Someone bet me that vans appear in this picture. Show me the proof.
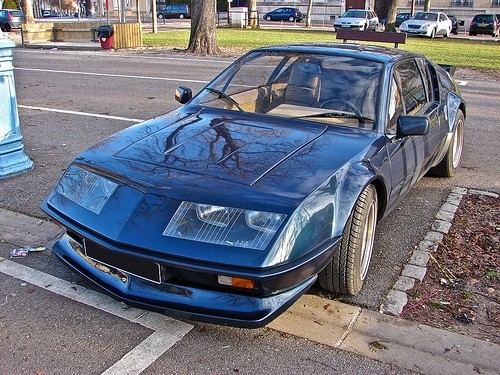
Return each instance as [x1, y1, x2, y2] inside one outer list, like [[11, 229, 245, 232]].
[[157, 4, 191, 19]]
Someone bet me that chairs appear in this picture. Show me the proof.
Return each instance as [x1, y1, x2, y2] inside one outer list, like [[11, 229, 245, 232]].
[[282, 56, 323, 107]]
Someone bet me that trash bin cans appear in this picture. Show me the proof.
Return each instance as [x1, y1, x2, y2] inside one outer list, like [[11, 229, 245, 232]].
[[96, 25, 114, 50]]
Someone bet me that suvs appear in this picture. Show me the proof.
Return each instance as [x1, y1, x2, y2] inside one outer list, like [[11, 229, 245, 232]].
[[469, 13, 500, 37]]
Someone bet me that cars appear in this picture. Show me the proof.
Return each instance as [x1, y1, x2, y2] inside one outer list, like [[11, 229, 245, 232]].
[[381, 13, 412, 27], [447, 15, 458, 35], [40, 42, 466, 329], [43, 11, 81, 18], [0, 9, 23, 32], [333, 9, 379, 32], [400, 12, 452, 39], [263, 7, 303, 22]]
